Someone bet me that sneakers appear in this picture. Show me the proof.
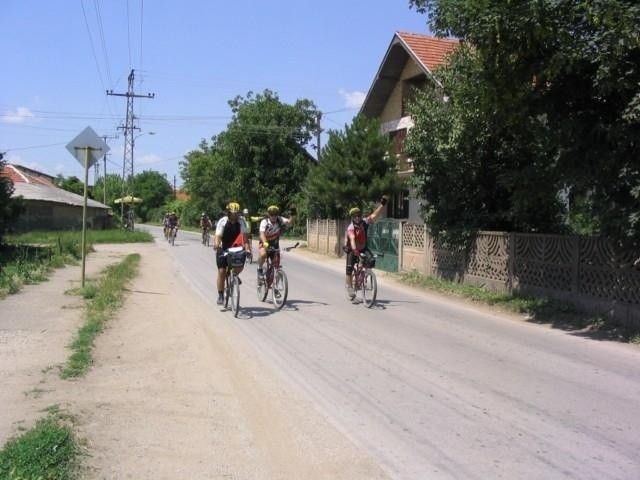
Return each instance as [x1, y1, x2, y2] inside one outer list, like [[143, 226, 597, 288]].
[[257, 269, 264, 279], [217, 290, 224, 304]]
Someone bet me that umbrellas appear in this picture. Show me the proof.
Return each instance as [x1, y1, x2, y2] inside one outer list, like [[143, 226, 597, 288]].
[[114, 195, 142, 204]]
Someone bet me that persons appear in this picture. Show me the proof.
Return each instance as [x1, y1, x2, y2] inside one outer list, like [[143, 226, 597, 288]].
[[200, 212, 213, 241], [239, 208, 265, 236], [344, 194, 390, 297], [213, 203, 253, 305], [162, 212, 180, 238], [256, 203, 297, 298]]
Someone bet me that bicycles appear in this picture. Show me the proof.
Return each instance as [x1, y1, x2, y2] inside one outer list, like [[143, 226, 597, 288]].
[[159, 222, 180, 247], [200, 225, 213, 248], [257, 240, 302, 307], [341, 247, 384, 310], [216, 242, 253, 318]]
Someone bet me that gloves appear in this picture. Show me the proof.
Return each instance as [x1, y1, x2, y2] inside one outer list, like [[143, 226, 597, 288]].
[[380, 195, 389, 205]]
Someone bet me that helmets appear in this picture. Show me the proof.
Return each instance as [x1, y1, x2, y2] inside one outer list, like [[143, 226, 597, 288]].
[[349, 207, 361, 216], [226, 202, 240, 214], [268, 205, 280, 214]]
